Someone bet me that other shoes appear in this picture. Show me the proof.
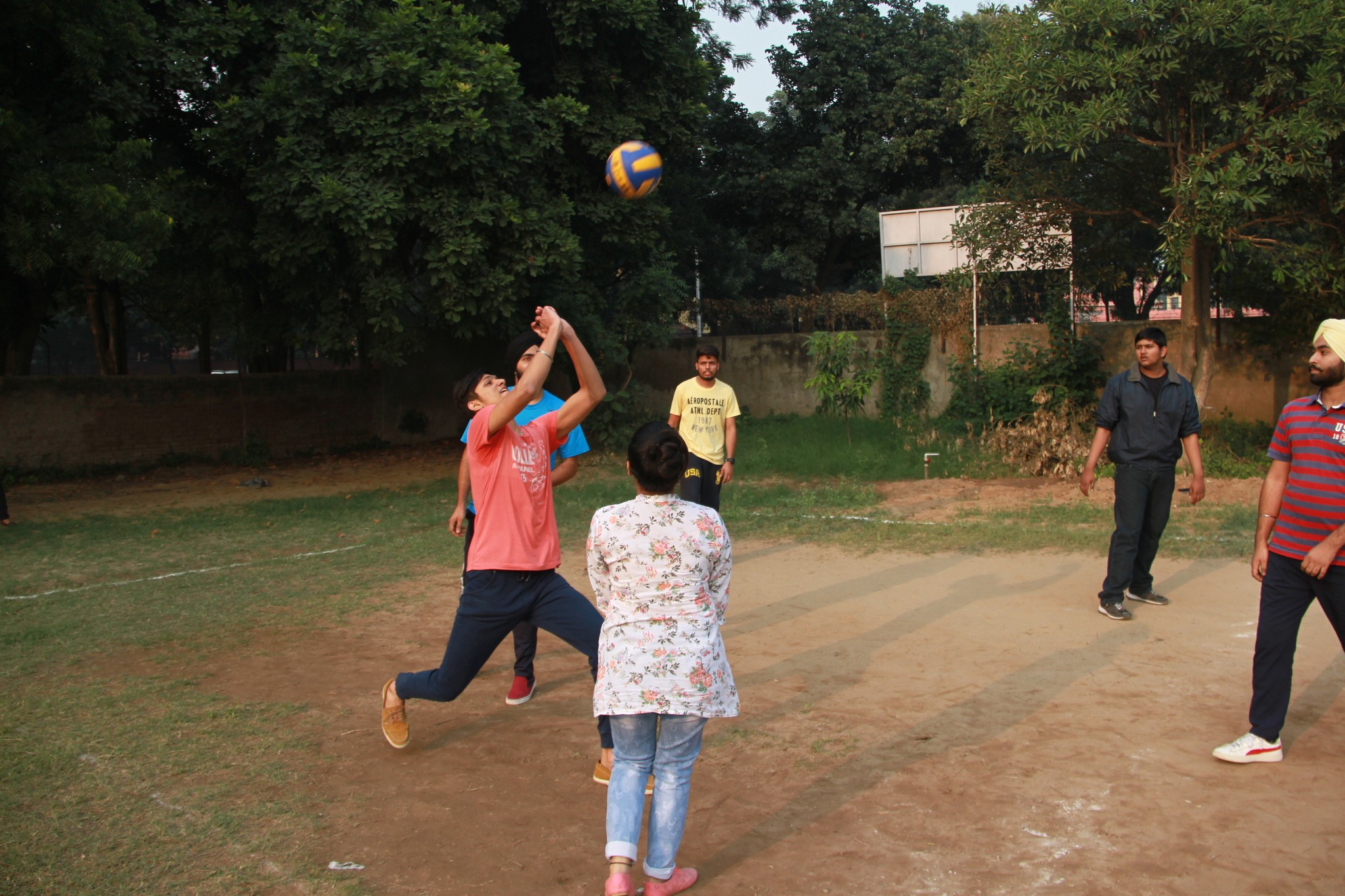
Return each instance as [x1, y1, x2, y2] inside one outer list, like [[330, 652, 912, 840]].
[[604, 872, 635, 895], [645, 867, 697, 896]]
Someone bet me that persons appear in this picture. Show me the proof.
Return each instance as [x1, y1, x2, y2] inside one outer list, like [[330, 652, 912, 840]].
[[586, 420, 742, 896], [447, 331, 590, 706], [379, 304, 665, 797], [1208, 315, 1345, 764], [1078, 326, 1206, 621], [668, 345, 742, 511]]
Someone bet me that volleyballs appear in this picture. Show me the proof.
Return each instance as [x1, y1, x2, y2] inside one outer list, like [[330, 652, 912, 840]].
[[605, 141, 663, 198]]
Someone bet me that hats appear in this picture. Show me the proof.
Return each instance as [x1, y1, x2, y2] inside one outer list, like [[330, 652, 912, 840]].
[[1313, 318, 1345, 362]]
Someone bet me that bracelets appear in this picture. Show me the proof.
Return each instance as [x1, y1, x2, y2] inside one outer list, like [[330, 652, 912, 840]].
[[1258, 514, 1276, 519], [536, 350, 554, 363]]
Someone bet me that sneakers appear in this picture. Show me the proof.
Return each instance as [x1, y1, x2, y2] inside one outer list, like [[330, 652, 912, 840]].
[[381, 678, 410, 749], [505, 674, 536, 704], [1126, 590, 1169, 605], [1212, 732, 1283, 764], [1098, 602, 1131, 620], [592, 760, 654, 794]]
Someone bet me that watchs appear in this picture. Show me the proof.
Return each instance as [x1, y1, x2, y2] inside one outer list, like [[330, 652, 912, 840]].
[[725, 457, 735, 465]]
